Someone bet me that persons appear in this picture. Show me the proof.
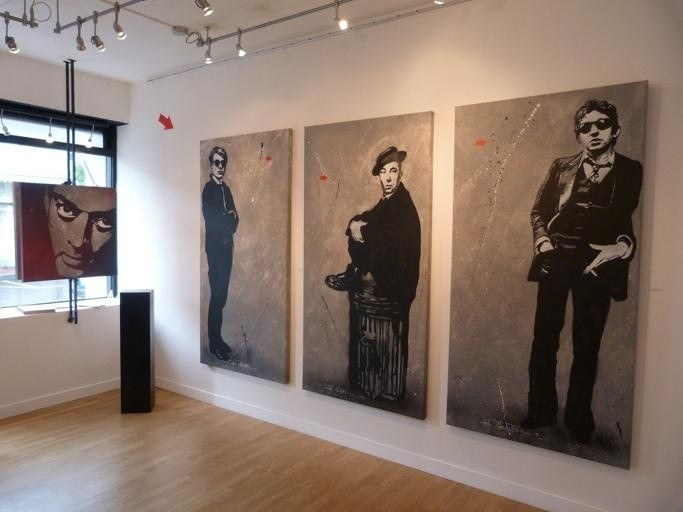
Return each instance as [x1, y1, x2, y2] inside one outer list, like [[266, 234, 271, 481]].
[[324, 145, 422, 311], [201, 145, 239, 364], [515, 99, 644, 439], [40, 185, 116, 280]]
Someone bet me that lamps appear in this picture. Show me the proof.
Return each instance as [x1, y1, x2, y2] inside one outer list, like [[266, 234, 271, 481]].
[[113, 2, 126, 40], [236, 30, 245, 57], [0, 109, 95, 149], [197, 33, 204, 47], [334, 3, 349, 30], [195, 1, 214, 17], [77, 15, 86, 52], [91, 11, 106, 52], [5, 11, 19, 53], [204, 37, 212, 65]]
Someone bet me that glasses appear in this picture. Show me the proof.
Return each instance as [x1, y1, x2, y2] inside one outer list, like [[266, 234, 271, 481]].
[[211, 160, 225, 166], [577, 119, 611, 135]]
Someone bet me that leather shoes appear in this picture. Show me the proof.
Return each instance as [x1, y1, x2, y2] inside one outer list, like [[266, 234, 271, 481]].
[[216, 341, 231, 361], [325, 264, 363, 290]]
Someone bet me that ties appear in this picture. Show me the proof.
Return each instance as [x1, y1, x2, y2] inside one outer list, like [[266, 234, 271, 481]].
[[589, 164, 600, 183]]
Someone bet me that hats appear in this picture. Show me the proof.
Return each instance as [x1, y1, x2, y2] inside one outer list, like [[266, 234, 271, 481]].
[[372, 147, 406, 176]]
